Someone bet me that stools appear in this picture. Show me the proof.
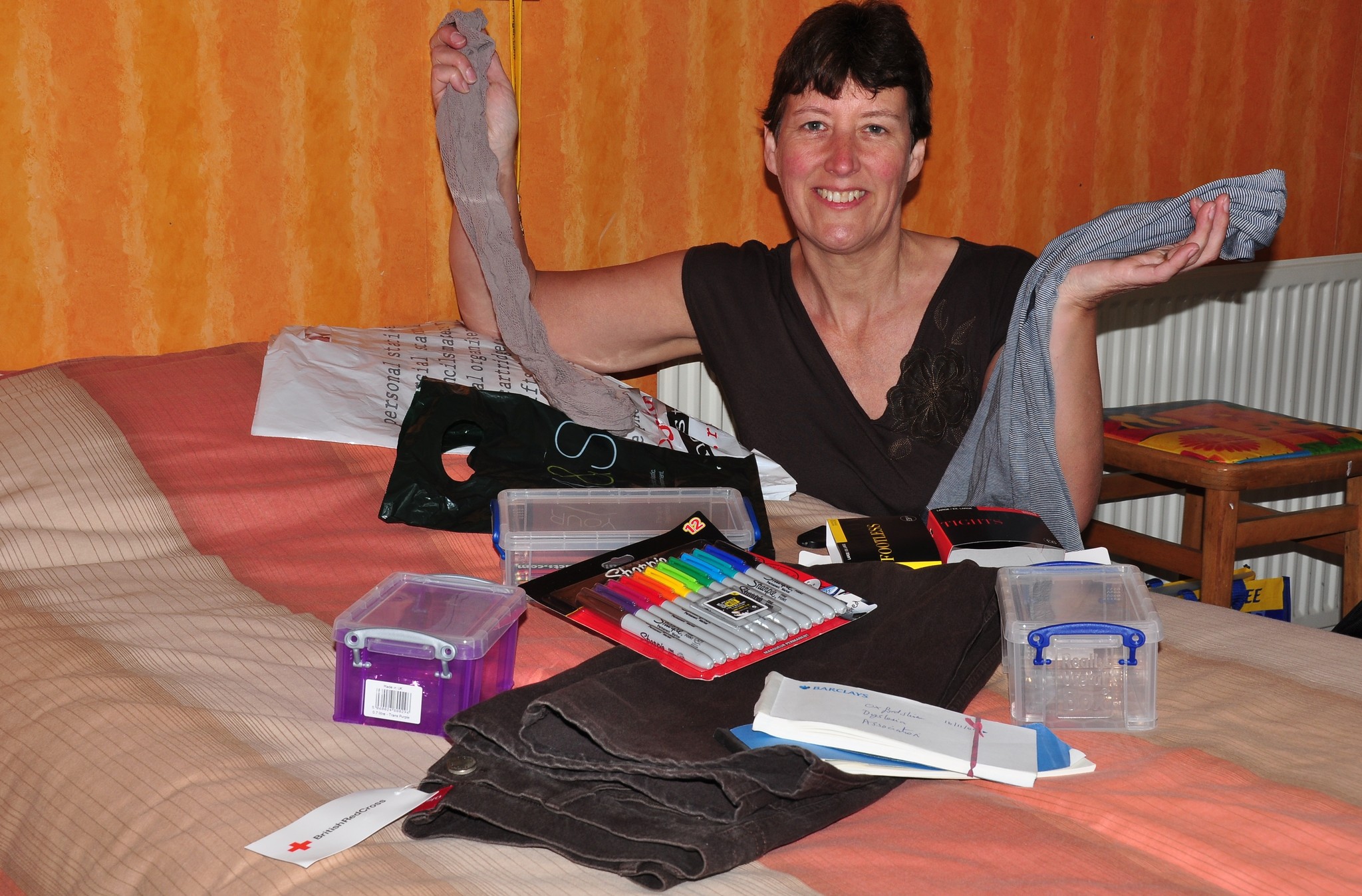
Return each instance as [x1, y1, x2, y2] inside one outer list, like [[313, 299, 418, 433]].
[[1081, 400, 1361, 620]]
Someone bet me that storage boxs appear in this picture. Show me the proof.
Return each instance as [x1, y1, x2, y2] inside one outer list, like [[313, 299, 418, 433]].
[[996, 561, 1163, 731], [823, 514, 944, 570], [332, 572, 529, 737], [929, 506, 1067, 568], [488, 486, 764, 589]]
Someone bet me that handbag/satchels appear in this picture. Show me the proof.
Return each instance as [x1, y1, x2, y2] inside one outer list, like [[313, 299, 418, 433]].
[[378, 375, 775, 559], [1145, 566, 1290, 630], [250, 320, 799, 502]]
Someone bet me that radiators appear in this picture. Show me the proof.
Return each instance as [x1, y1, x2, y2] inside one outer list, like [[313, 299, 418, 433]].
[[655, 252, 1362, 627]]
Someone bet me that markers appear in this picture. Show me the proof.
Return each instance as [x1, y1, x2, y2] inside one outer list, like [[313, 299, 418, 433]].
[[574, 540, 849, 668]]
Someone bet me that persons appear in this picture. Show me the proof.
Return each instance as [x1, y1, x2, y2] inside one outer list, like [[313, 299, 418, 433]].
[[429, 0, 1229, 533]]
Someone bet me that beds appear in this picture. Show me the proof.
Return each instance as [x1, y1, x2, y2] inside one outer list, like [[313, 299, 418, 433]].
[[0, 338, 1362, 896]]
